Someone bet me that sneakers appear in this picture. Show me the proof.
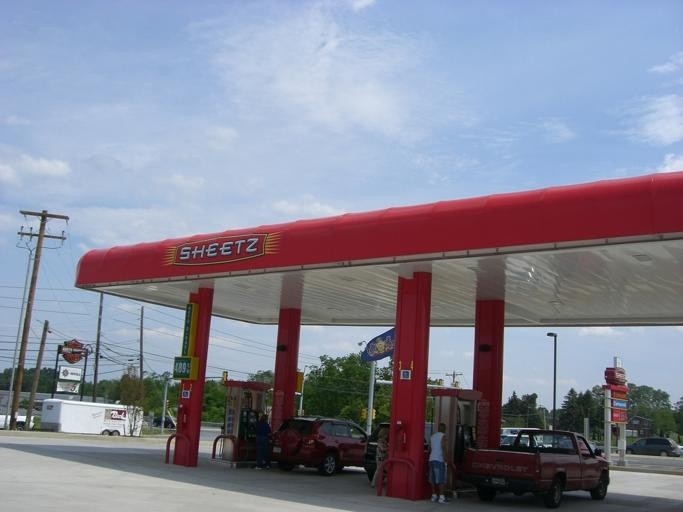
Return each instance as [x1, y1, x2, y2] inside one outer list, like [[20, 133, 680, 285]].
[[431, 493, 448, 504]]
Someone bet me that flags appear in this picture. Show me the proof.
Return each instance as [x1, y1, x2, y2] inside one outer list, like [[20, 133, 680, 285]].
[[361, 327, 395, 362]]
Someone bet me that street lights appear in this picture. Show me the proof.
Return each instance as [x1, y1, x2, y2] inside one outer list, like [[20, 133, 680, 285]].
[[548, 333, 556, 448], [358, 340, 375, 436]]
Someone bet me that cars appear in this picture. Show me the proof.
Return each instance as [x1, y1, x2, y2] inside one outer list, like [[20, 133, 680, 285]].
[[153, 415, 177, 428], [626, 437, 681, 457]]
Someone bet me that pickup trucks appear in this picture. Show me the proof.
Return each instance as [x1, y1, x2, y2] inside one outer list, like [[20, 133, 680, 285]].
[[0, 415, 36, 430]]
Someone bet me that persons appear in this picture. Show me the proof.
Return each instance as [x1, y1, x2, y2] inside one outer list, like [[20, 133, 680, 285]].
[[427, 423, 448, 504], [370, 427, 390, 488], [254, 414, 272, 470]]
